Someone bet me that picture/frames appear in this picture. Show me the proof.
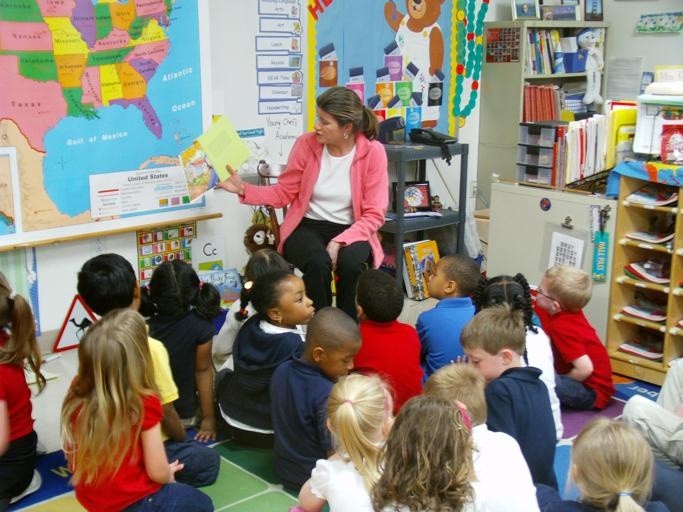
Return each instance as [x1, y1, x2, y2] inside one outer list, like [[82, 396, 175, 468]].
[[392, 180, 432, 213], [511, 0, 541, 20], [584, 0, 603, 21]]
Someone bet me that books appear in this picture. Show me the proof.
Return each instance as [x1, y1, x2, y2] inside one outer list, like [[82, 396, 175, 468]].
[[618, 335, 664, 361], [625, 230, 674, 245], [400, 238, 441, 300], [631, 63, 683, 167], [524, 29, 566, 76], [622, 258, 670, 287], [177, 114, 252, 205], [550, 101, 642, 188], [619, 296, 667, 323], [524, 83, 561, 121], [625, 181, 678, 206]]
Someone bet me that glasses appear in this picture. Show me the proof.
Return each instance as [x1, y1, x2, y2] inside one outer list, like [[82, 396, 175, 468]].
[[535, 286, 564, 311]]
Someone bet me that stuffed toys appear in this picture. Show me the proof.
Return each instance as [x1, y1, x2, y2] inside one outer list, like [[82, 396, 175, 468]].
[[578, 30, 604, 105]]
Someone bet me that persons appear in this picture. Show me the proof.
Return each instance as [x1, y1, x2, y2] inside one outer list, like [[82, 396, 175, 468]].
[[474, 273, 565, 443], [268, 306, 364, 491], [58, 307, 221, 512], [415, 253, 482, 387], [0, 268, 47, 512], [214, 85, 389, 326], [211, 248, 308, 371], [533, 416, 669, 512], [288, 373, 396, 512], [75, 252, 221, 488], [348, 268, 425, 419], [368, 394, 482, 512], [138, 257, 221, 444], [424, 362, 541, 512], [459, 302, 561, 493], [215, 269, 316, 450], [530, 264, 615, 411], [621, 357, 683, 471], [518, 4, 535, 17]]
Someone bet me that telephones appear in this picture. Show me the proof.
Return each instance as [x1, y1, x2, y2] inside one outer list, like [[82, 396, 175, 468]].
[[409, 128, 458, 166]]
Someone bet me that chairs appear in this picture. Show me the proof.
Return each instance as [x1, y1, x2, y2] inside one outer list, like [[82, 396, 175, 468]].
[[260, 175, 298, 274]]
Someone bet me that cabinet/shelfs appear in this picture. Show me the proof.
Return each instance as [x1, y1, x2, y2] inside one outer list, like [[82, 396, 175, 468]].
[[377, 140, 472, 293], [481, 18, 610, 195], [605, 158, 683, 388]]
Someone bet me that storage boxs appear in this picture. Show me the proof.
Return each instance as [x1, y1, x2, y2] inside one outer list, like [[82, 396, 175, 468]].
[[516, 121, 558, 148], [516, 162, 553, 186], [515, 143, 556, 169]]
